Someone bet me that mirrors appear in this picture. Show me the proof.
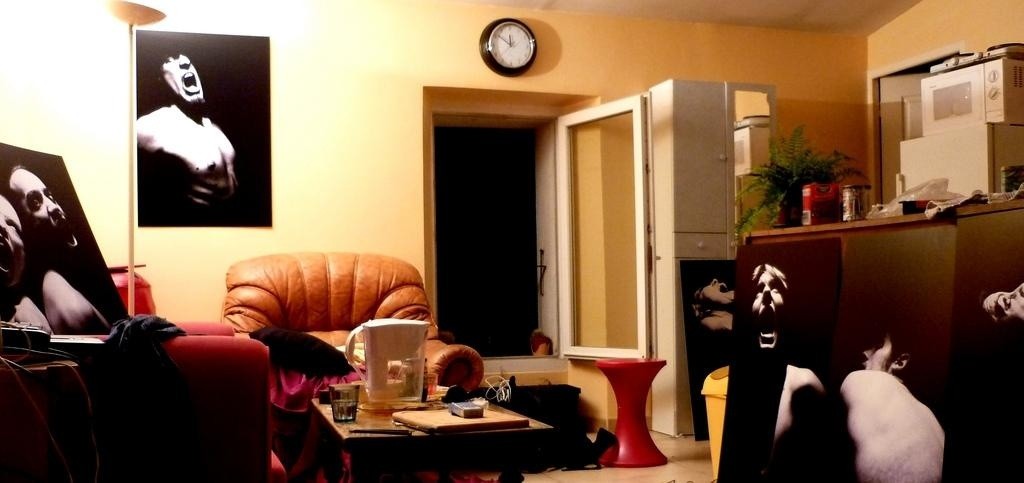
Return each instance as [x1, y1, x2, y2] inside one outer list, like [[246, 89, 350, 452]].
[[733, 88, 770, 253]]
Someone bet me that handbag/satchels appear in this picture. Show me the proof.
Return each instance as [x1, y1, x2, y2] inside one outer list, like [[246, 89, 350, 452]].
[[469, 382, 592, 472]]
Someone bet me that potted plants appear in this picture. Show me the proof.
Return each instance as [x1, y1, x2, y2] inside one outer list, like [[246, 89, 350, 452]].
[[726, 121, 867, 244]]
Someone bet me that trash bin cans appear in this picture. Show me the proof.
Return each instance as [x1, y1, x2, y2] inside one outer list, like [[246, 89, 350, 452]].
[[701, 365, 729, 481]]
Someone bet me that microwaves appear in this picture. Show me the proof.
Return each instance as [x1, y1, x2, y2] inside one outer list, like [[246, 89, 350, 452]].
[[734, 127, 772, 175], [919, 59, 1024, 137]]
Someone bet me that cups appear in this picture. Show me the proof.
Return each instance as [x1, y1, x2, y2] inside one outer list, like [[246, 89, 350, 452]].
[[328, 384, 360, 422]]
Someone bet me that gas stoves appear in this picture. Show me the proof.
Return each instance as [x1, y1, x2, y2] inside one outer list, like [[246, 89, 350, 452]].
[[928, 42, 1024, 73], [735, 114, 771, 131]]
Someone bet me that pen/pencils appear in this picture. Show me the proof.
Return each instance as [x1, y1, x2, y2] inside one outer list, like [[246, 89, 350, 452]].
[[349, 429, 412, 435]]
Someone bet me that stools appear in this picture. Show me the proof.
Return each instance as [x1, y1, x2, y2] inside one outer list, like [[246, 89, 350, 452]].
[[593, 355, 669, 467]]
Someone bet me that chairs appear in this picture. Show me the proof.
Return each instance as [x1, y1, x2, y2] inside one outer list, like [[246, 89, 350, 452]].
[[111, 268, 272, 476], [226, 252, 483, 440]]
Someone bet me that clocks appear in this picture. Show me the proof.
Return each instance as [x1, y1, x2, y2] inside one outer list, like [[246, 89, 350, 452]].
[[481, 18, 537, 77]]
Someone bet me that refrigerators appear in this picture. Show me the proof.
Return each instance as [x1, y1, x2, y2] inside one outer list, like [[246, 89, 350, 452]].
[[735, 172, 774, 232], [896, 124, 1024, 203]]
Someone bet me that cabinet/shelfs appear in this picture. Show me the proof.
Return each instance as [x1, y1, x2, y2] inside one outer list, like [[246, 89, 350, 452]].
[[650, 78, 777, 438]]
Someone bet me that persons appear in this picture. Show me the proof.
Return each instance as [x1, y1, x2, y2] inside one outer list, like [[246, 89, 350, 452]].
[[980, 282, 1024, 329], [6, 164, 111, 335], [749, 263, 827, 483], [691, 278, 735, 331], [137, 47, 239, 226], [0, 193, 55, 336], [837, 333, 944, 483]]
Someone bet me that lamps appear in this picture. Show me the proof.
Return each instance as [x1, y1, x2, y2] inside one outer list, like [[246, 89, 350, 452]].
[[103, 0, 165, 319]]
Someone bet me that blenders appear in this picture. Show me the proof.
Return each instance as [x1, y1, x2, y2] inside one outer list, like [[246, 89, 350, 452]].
[[344, 318, 430, 408]]
